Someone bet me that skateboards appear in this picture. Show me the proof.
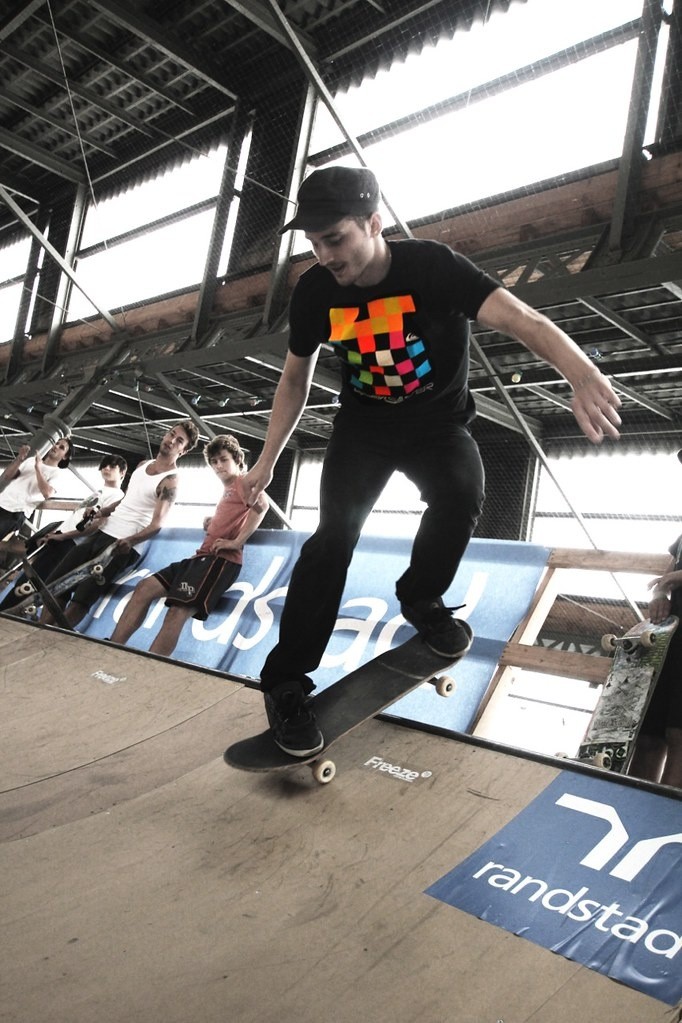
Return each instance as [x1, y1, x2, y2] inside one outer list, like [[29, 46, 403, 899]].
[[0, 542, 51, 582], [574, 613, 676, 779], [223, 614, 474, 785], [5, 540, 120, 617], [11, 553, 75, 634]]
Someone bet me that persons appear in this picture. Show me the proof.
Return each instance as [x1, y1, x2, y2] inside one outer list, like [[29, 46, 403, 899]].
[[38, 419, 199, 630], [103, 434, 269, 657], [0, 455, 127, 615], [627, 534, 682, 788], [0, 438, 74, 542], [238, 167, 622, 759]]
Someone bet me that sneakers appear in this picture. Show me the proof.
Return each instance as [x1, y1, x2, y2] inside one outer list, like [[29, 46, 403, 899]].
[[264, 683, 324, 757], [400, 596, 472, 658]]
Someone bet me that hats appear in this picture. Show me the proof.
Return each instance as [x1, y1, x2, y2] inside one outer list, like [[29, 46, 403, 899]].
[[278, 166, 380, 236]]
